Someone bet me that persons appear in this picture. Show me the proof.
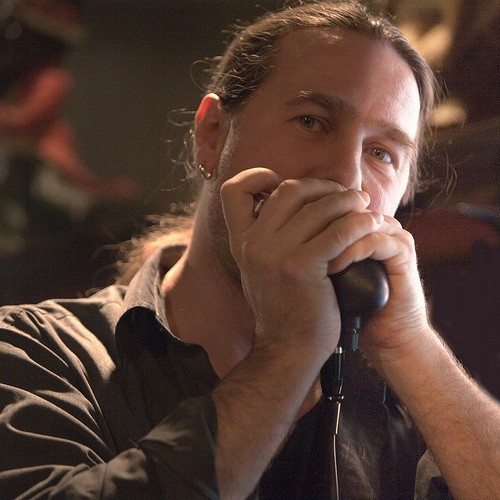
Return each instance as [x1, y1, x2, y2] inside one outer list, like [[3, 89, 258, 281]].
[[0, 2, 499, 500]]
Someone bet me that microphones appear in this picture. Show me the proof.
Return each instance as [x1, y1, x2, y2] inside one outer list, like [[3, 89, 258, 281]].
[[327, 257, 390, 405]]
